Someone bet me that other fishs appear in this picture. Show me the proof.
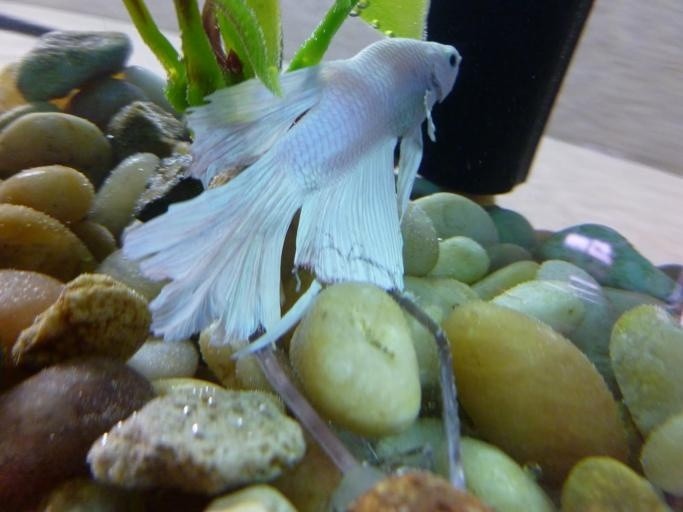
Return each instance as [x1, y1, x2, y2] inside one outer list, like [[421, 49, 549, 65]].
[[120, 37, 463, 350]]
